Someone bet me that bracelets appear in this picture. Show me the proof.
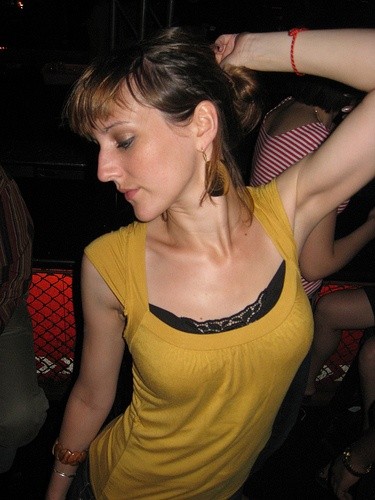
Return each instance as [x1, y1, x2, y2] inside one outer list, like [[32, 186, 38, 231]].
[[52, 469, 77, 480], [288, 28, 308, 75], [52, 437, 87, 466]]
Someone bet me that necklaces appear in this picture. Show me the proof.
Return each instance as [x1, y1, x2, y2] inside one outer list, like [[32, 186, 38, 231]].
[[314, 107, 321, 123]]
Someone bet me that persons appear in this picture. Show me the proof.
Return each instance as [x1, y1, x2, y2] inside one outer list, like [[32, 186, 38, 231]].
[[0, 19, 375, 500]]
